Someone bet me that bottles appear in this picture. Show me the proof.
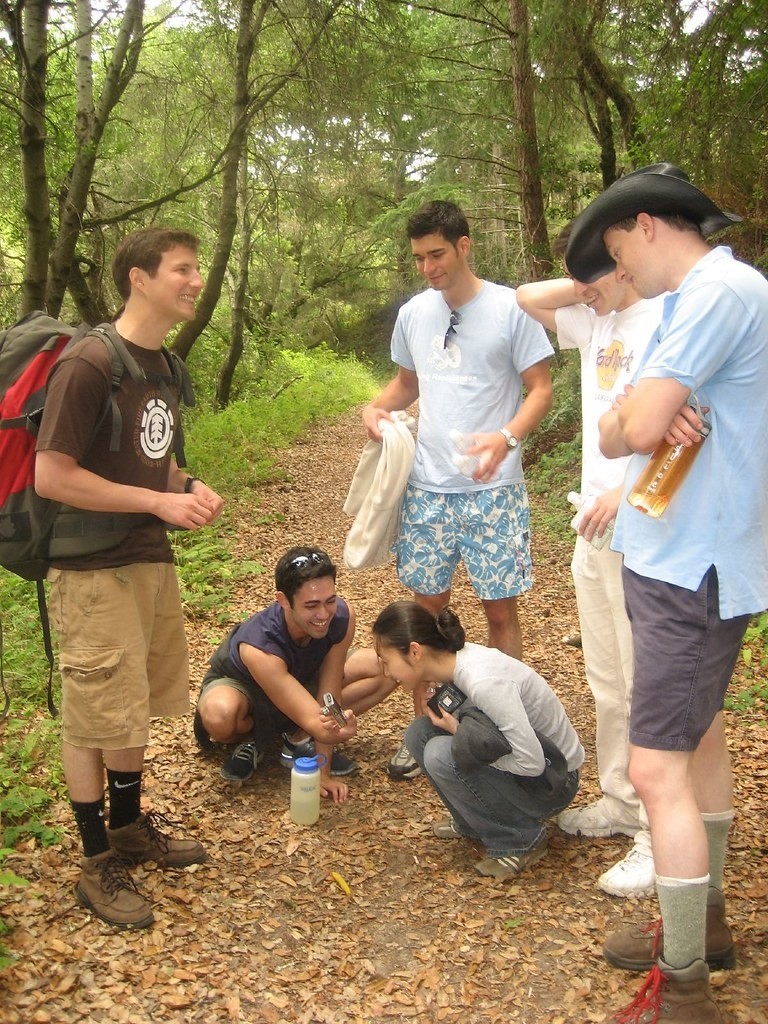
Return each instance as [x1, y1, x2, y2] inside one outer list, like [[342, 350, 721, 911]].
[[448, 430, 480, 458], [567, 492, 615, 526], [451, 454, 504, 482], [290, 753, 327, 825], [627, 395, 711, 518]]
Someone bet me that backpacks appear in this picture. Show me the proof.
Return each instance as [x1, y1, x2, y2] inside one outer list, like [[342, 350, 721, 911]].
[[0, 310, 195, 580]]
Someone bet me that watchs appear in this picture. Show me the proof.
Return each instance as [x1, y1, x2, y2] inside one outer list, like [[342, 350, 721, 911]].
[[500, 427, 518, 449]]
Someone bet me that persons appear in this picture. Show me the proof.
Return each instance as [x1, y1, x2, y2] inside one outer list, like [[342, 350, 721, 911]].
[[564, 161, 768, 1024], [196, 545, 401, 802], [35, 231, 226, 931], [516, 217, 676, 899], [363, 202, 556, 780], [372, 601, 585, 878]]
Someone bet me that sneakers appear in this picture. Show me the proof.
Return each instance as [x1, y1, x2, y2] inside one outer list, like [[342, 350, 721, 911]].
[[74, 848, 154, 930], [598, 849, 656, 898], [106, 809, 207, 866], [433, 819, 462, 838], [221, 742, 264, 782], [599, 955, 723, 1024], [557, 796, 642, 838], [387, 742, 422, 779], [281, 732, 362, 776], [474, 835, 549, 877], [603, 887, 736, 970]]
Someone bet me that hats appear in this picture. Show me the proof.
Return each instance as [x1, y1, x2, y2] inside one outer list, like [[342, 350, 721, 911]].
[[565, 163, 743, 284]]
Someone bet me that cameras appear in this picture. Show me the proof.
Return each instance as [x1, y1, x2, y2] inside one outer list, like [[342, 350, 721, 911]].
[[427, 681, 467, 718], [322, 693, 346, 729]]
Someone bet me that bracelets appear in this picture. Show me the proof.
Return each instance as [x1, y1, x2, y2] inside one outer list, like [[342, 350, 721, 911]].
[[185, 477, 207, 493]]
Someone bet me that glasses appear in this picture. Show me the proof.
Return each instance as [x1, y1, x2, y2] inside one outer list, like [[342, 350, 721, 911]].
[[442, 310, 462, 350], [282, 552, 328, 582]]
